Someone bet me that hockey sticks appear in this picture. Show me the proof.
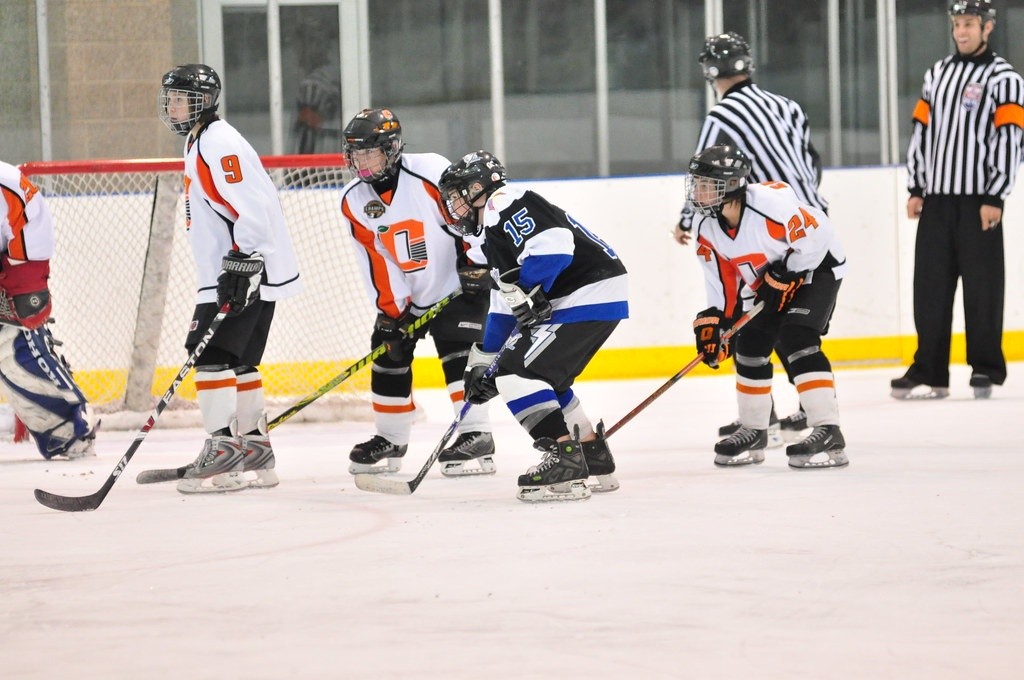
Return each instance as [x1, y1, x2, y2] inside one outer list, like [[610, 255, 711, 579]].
[[134, 289, 464, 484], [602, 302, 770, 437], [352, 313, 526, 496], [34, 300, 233, 514]]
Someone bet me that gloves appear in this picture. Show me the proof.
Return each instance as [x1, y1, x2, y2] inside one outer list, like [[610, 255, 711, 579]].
[[216, 250, 263, 316], [752, 259, 810, 316], [492, 269, 552, 326], [693, 306, 736, 369], [464, 342, 499, 404], [0, 254, 52, 330]]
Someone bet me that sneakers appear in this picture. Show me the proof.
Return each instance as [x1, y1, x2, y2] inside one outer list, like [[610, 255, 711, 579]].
[[438, 432, 496, 476], [349, 435, 408, 474], [243, 412, 280, 487], [176, 416, 244, 493], [580, 419, 621, 492], [786, 424, 850, 468], [779, 412, 809, 431], [714, 425, 768, 465], [970, 373, 992, 398], [60, 437, 96, 456], [517, 424, 592, 501], [719, 410, 785, 448], [890, 363, 950, 399]]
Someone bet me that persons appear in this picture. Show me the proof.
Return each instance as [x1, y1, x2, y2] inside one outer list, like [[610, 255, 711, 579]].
[[285, 17, 343, 154], [337, 107, 495, 464], [0, 160, 102, 461], [159, 63, 300, 479], [674, 32, 845, 456], [438, 149, 630, 487], [889, 0, 1024, 389]]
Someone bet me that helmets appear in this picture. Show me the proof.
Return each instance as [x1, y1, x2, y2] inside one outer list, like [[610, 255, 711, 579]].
[[686, 145, 752, 216], [948, 0, 997, 24], [343, 108, 406, 184], [162, 64, 221, 136], [698, 30, 755, 84], [439, 150, 511, 236]]
